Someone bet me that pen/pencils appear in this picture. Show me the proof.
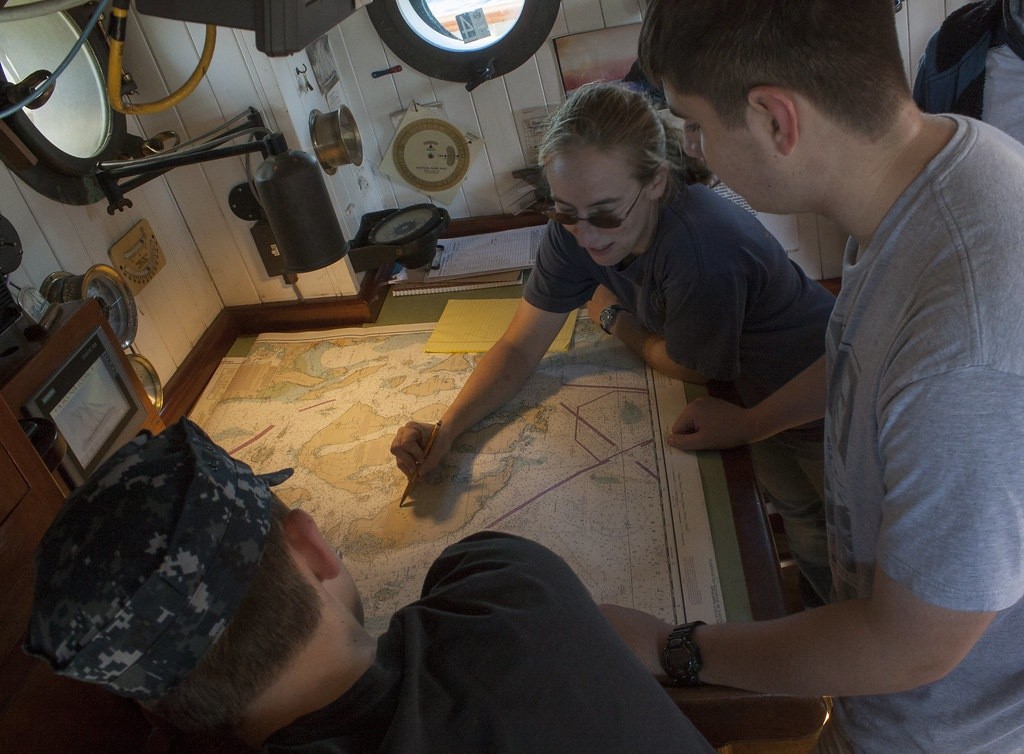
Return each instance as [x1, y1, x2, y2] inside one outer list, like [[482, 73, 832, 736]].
[[399, 419, 443, 506]]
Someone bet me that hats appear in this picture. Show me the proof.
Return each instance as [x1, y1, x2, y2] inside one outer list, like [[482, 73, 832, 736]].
[[24, 417, 295, 702]]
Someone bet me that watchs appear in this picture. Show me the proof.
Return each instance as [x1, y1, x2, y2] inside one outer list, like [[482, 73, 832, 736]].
[[599, 304, 632, 335], [660, 622, 708, 690]]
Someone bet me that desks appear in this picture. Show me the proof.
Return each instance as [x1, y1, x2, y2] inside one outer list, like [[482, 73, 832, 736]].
[[186, 220, 828, 754]]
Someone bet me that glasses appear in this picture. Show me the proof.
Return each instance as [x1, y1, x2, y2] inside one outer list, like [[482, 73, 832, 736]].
[[540, 179, 643, 229]]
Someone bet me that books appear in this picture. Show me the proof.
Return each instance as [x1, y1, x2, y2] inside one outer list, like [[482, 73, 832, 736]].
[[388, 224, 548, 297]]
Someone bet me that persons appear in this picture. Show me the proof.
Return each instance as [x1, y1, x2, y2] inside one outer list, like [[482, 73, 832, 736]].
[[390, 82, 842, 617], [592, 1, 1024, 753], [912, 1, 1024, 143], [14, 418, 725, 754]]
